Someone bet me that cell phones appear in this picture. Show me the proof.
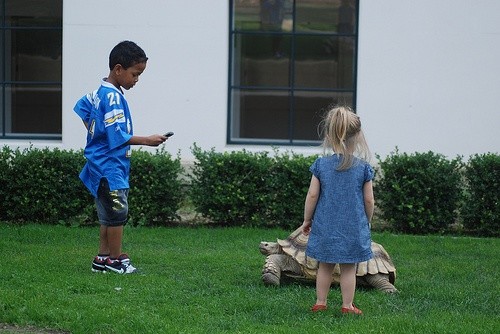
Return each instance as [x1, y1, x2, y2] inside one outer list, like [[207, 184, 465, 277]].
[[164, 132, 174, 137]]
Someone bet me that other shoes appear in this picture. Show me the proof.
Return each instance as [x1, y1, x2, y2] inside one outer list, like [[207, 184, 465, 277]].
[[342, 303, 363, 314], [312, 305, 326, 310]]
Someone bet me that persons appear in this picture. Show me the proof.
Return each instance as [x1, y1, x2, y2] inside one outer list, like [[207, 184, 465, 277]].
[[73, 40, 168, 275], [301, 106, 375, 315]]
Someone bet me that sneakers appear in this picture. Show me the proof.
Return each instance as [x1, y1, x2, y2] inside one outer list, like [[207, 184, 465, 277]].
[[91, 255, 110, 272], [103, 254, 136, 274]]
